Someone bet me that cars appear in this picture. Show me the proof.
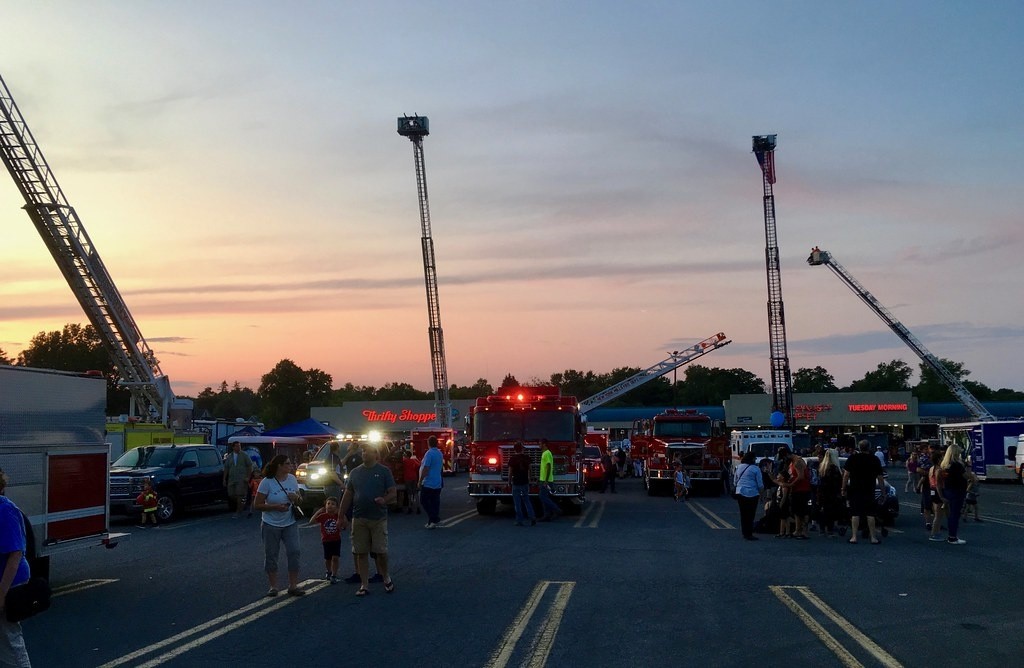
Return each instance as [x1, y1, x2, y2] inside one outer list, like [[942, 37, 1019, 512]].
[[776, 456, 900, 517]]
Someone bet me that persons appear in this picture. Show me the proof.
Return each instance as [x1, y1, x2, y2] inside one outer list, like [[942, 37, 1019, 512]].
[[674, 463, 693, 503], [507, 443, 538, 527], [137, 481, 160, 529], [307, 434, 444, 597], [536, 439, 561, 522], [248, 468, 263, 518], [599, 444, 643, 494], [223, 441, 253, 518], [0, 468, 32, 668], [253, 454, 305, 596], [733, 438, 983, 544]]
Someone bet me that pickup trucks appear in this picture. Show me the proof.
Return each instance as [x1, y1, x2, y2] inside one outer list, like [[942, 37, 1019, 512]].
[[292, 435, 444, 515], [109, 444, 227, 524]]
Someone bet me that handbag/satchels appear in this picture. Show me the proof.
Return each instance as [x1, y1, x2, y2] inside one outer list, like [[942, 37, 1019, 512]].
[[4, 555, 52, 622], [936, 469, 956, 505], [292, 506, 305, 521], [731, 487, 739, 500]]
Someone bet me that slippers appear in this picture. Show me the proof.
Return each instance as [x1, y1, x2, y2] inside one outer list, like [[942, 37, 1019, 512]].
[[355, 586, 368, 597], [384, 577, 394, 593]]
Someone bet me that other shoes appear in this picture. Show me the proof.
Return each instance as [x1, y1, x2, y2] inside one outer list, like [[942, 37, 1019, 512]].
[[773, 532, 792, 539], [288, 584, 306, 596], [424, 522, 436, 529], [344, 573, 360, 583], [791, 534, 808, 542], [151, 523, 159, 528], [743, 533, 758, 540], [871, 539, 882, 544], [325, 570, 332, 580], [535, 516, 551, 522], [368, 574, 384, 583], [847, 537, 857, 544], [974, 518, 983, 522], [947, 536, 966, 544], [963, 519, 967, 522], [926, 523, 933, 529], [929, 534, 945, 541], [267, 587, 278, 597], [136, 523, 146, 529], [330, 575, 341, 584]]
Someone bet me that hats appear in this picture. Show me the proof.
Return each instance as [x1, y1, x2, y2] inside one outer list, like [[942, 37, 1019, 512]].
[[360, 443, 378, 453], [778, 448, 791, 460], [877, 446, 881, 448]]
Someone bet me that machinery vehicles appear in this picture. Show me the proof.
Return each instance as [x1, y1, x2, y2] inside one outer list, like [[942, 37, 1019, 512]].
[[751, 131, 811, 450], [396, 112, 471, 474]]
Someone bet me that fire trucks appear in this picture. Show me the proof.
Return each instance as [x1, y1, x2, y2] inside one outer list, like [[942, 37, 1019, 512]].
[[630, 408, 733, 496], [581, 426, 615, 482], [463, 332, 733, 514]]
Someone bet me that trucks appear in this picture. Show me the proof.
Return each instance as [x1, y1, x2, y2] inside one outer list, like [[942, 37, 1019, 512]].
[[731, 427, 793, 474]]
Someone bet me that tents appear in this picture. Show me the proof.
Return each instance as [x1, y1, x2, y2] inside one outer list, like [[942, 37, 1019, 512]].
[[216, 418, 341, 452]]
